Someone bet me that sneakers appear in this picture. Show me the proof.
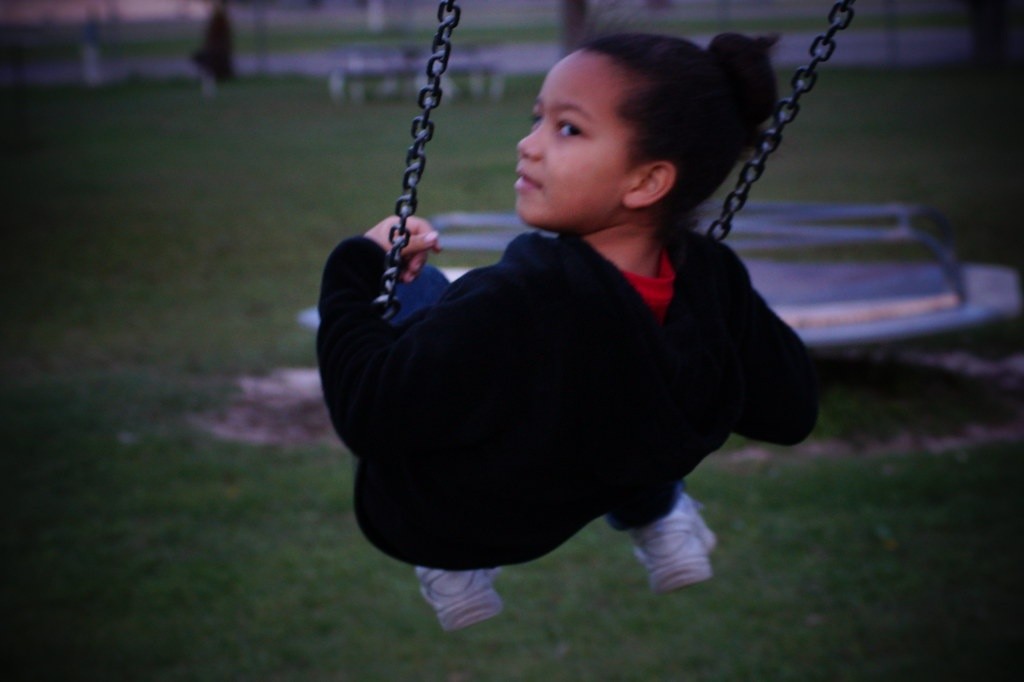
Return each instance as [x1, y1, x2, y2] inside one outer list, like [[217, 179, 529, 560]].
[[415, 566, 502, 633], [630, 492, 716, 593]]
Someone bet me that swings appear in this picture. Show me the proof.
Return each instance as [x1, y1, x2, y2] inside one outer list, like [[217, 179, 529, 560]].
[[372, 0, 853, 511]]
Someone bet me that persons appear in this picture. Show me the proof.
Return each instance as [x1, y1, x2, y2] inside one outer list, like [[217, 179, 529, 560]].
[[316, 33, 822, 630]]
